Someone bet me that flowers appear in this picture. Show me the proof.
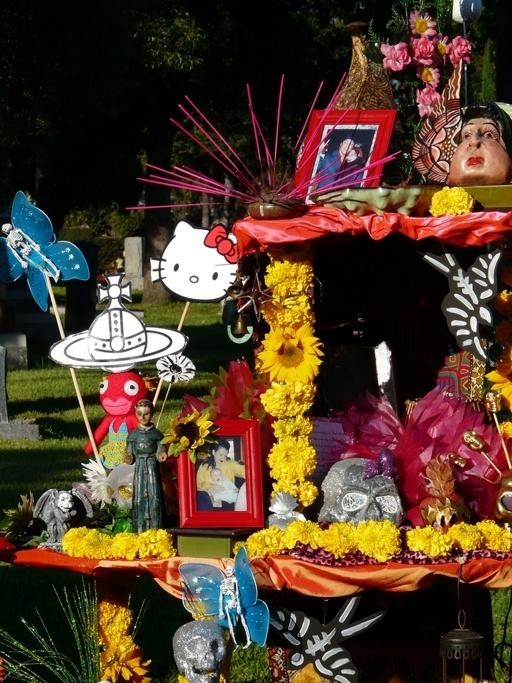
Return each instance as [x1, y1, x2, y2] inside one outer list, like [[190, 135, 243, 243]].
[[57, 517, 511, 567], [367, 0, 473, 131], [77, 455, 141, 513], [82, 569, 155, 682], [261, 252, 328, 507], [426, 184, 474, 216], [161, 408, 224, 466], [480, 362, 512, 449]]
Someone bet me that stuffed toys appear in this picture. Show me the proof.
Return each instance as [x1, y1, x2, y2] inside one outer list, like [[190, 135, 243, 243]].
[[78, 373, 148, 474]]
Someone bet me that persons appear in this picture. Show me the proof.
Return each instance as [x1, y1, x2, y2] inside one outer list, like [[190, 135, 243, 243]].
[[315, 133, 351, 188], [196, 439, 246, 511], [337, 147, 363, 187], [208, 468, 240, 506], [121, 399, 168, 531], [445, 102, 511, 183]]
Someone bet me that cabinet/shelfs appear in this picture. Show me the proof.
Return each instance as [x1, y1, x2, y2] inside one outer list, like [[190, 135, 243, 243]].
[[2, 202, 511, 682]]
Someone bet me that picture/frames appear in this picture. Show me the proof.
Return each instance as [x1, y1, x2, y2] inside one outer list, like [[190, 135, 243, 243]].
[[174, 416, 267, 531], [290, 105, 403, 205]]
